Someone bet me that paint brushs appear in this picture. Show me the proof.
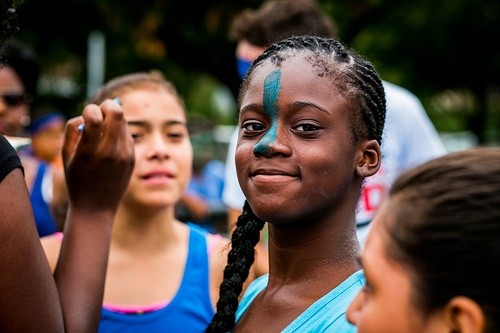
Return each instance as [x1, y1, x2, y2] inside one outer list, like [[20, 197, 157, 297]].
[[78, 97, 122, 132]]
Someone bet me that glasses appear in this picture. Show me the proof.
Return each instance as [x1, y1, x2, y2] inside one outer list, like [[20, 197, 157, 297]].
[[0, 94, 26, 108]]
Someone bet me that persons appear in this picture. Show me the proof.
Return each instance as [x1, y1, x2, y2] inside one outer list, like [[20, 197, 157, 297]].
[[0, 0, 448, 333], [345, 147, 500, 333]]
